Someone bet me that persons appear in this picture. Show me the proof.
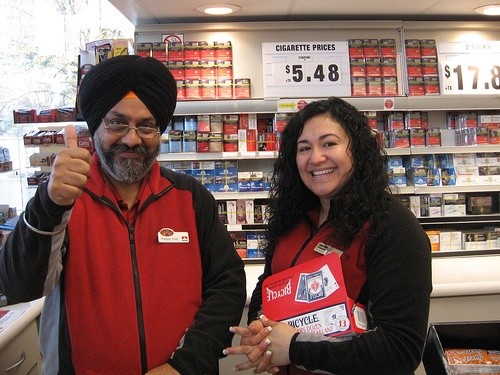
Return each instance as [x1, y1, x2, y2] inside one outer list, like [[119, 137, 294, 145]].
[[222, 96, 433, 375], [0, 55, 247, 375]]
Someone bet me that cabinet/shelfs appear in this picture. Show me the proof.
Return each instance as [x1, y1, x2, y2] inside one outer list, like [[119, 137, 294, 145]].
[[132, 20, 500, 306]]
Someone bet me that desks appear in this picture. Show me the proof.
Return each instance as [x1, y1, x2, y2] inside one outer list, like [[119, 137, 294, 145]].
[[0, 210, 23, 231]]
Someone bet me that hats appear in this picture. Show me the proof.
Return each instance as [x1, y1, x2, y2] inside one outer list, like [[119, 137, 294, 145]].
[[79, 55, 178, 137]]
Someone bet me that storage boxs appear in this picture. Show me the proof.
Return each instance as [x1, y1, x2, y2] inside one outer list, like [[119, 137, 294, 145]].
[[363, 111, 442, 148], [158, 113, 293, 153], [421, 320, 500, 375], [8, 207, 17, 218], [161, 160, 279, 192], [421, 226, 500, 252], [0, 212, 5, 225], [0, 161, 12, 172], [3, 210, 8, 219], [137, 41, 252, 100], [392, 192, 493, 217], [217, 199, 274, 224], [230, 231, 270, 258], [12, 106, 95, 187], [447, 112, 500, 146], [260, 252, 368, 338], [405, 39, 440, 96], [347, 38, 397, 97], [385, 153, 500, 187]]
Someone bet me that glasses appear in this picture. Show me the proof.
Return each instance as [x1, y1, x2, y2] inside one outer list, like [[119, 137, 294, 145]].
[[100, 115, 158, 135]]
